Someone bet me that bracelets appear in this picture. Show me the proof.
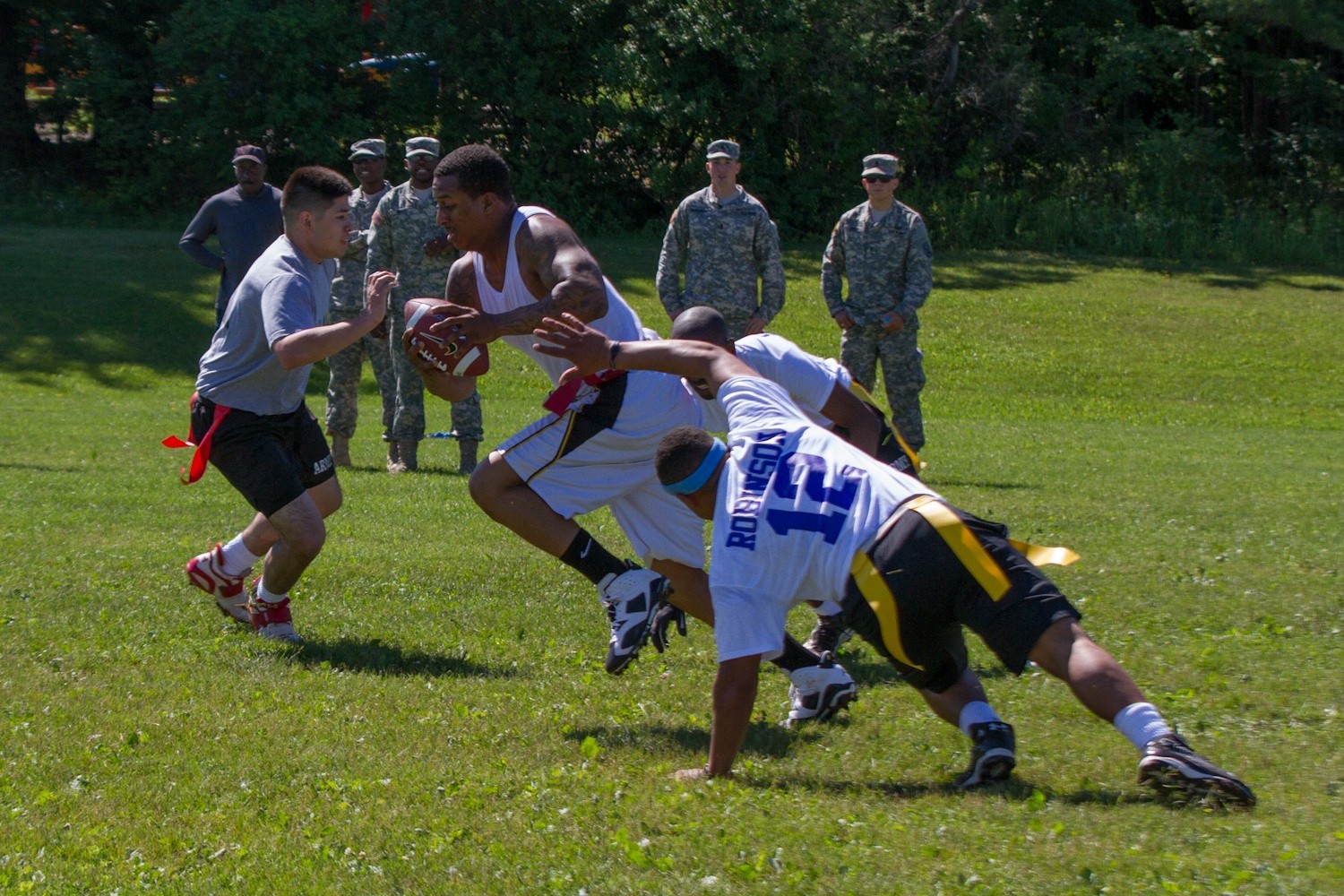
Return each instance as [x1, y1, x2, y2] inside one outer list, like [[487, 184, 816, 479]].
[[609, 340, 621, 371]]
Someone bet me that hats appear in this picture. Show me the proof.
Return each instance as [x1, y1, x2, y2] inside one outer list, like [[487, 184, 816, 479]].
[[706, 139, 740, 159], [861, 154, 898, 177], [405, 136, 440, 157], [348, 138, 386, 161], [231, 144, 264, 164]]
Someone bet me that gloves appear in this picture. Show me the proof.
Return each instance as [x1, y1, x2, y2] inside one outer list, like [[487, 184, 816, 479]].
[[647, 603, 688, 654]]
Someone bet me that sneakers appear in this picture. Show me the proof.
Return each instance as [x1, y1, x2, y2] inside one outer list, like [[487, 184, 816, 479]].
[[778, 652, 859, 731], [803, 618, 853, 654], [605, 559, 670, 675], [1137, 733, 1256, 807], [184, 541, 254, 625], [247, 576, 303, 647], [954, 721, 1018, 788]]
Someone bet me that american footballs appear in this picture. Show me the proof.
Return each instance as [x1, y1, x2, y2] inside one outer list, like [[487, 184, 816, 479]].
[[403, 296, 491, 380]]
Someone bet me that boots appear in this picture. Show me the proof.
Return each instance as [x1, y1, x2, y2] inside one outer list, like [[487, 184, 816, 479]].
[[330, 430, 351, 469], [456, 440, 478, 475], [386, 439, 418, 473]]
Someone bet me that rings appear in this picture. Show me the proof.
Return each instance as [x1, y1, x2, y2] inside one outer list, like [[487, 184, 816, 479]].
[[566, 338, 574, 348], [841, 324, 844, 327]]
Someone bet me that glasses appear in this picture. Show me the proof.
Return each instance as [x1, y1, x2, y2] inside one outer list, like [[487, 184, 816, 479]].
[[408, 155, 435, 162], [352, 157, 376, 164], [866, 175, 890, 183], [233, 164, 258, 173]]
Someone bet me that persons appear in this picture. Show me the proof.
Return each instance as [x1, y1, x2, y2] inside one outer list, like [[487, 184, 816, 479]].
[[670, 307, 1038, 672], [532, 311, 1258, 809], [430, 145, 860, 735], [180, 135, 483, 475], [657, 140, 786, 339], [185, 167, 396, 643], [821, 154, 933, 455]]
